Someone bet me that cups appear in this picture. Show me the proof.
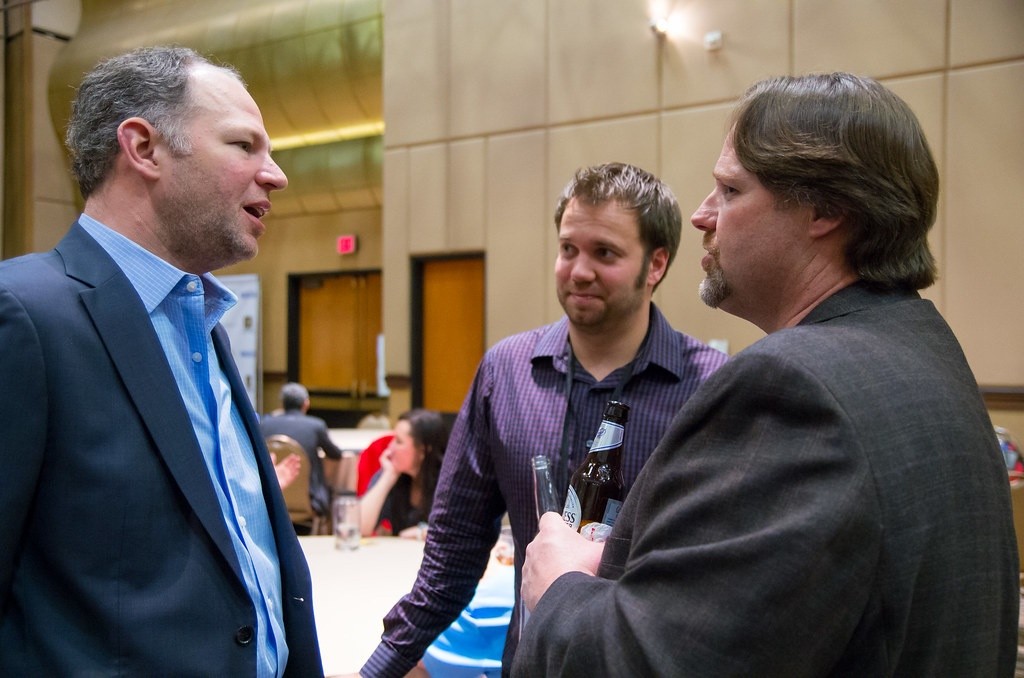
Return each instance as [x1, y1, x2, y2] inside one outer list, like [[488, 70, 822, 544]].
[[495, 525, 516, 568]]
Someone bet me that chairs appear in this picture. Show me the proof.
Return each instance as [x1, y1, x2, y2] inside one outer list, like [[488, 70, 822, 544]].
[[266, 433, 316, 523], [355, 410, 393, 430]]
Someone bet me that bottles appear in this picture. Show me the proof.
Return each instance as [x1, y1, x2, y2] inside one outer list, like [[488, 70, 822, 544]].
[[331, 491, 360, 550], [561, 400, 629, 535], [519, 454, 562, 642]]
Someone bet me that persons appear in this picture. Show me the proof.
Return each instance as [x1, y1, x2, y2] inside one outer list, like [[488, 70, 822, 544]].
[[354, 409, 452, 539], [0, 43, 324, 677], [353, 159, 729, 677], [249, 380, 342, 537], [503, 71, 1020, 678]]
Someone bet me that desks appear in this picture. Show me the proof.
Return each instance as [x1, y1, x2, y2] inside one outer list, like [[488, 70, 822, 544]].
[[297, 535, 425, 678], [320, 428, 393, 493]]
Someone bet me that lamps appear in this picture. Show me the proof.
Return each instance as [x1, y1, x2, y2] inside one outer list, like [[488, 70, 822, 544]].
[[647, 0, 668, 36]]
